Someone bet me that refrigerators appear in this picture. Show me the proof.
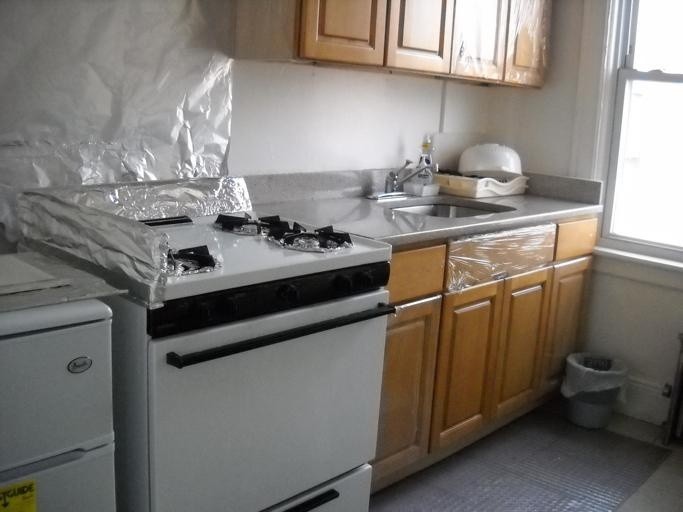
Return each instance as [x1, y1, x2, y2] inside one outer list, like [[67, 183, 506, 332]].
[[0, 298, 115, 512]]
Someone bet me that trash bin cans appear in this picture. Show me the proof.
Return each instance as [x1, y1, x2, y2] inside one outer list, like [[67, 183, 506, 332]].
[[566, 353, 629, 428]]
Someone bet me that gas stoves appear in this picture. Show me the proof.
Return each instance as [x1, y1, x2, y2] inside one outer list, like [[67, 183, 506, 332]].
[[135, 207, 392, 287]]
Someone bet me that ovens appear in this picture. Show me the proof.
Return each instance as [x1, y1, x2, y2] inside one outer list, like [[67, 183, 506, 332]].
[[145, 260, 393, 512]]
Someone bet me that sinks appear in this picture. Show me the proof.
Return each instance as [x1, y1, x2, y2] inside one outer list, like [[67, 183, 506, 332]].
[[375, 192, 518, 219]]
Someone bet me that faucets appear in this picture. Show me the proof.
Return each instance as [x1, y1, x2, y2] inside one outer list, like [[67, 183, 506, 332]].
[[386, 159, 440, 195], [384, 206, 427, 237]]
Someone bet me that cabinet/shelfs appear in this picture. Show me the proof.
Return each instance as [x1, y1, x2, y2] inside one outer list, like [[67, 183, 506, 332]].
[[299, 0, 553, 87], [430, 218, 558, 465], [370, 220, 449, 495], [537, 219, 598, 410]]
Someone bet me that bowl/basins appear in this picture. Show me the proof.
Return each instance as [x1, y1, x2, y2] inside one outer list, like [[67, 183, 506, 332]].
[[457, 143, 523, 180]]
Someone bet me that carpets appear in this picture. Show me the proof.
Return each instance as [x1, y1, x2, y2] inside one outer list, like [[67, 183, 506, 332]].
[[369, 410, 674, 512]]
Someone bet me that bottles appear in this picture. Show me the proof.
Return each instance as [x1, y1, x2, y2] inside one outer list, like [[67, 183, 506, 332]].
[[417, 132, 435, 166]]
